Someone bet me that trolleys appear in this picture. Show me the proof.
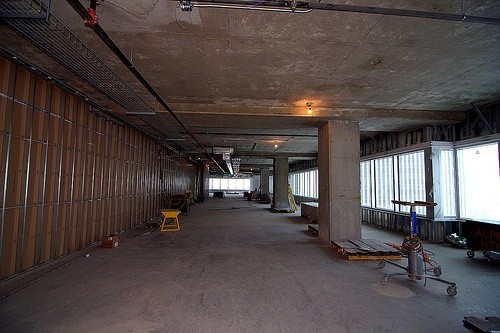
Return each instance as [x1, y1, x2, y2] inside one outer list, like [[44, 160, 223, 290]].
[[463, 229, 500, 257]]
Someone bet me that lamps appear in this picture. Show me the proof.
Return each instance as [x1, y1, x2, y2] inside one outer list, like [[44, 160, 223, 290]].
[[306, 102, 313, 114], [274, 140, 278, 147]]
